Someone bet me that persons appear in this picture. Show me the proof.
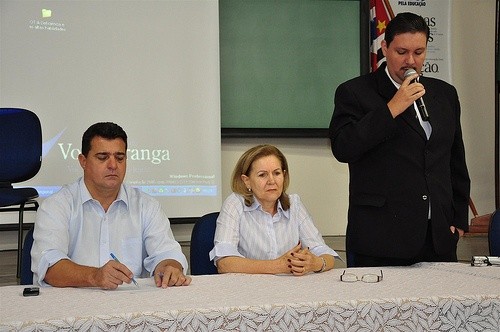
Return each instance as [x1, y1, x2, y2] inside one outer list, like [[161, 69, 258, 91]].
[[329, 12, 470, 269], [30, 122, 192, 290], [209, 145, 345, 277]]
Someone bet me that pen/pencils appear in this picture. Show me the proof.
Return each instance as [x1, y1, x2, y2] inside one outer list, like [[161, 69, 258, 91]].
[[110, 253, 141, 288]]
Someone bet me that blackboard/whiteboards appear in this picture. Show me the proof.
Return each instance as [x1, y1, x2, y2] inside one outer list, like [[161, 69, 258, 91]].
[[220, 1, 370, 138]]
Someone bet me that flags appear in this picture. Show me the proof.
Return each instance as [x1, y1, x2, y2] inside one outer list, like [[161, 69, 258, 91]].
[[370, 1, 395, 72]]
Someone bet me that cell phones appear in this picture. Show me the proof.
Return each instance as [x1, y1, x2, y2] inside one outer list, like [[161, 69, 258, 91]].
[[23, 288, 39, 296]]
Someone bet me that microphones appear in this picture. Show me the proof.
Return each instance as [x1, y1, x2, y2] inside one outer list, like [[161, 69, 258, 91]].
[[404, 69, 429, 121]]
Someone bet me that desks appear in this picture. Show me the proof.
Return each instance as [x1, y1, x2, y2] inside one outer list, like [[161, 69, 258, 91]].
[[0, 262, 500, 332]]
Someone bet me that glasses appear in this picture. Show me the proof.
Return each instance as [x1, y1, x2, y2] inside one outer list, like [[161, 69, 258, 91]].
[[340, 270, 383, 283]]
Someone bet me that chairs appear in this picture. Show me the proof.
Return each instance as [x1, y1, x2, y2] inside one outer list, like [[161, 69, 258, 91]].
[[0, 108, 42, 278], [190, 212, 222, 275]]
